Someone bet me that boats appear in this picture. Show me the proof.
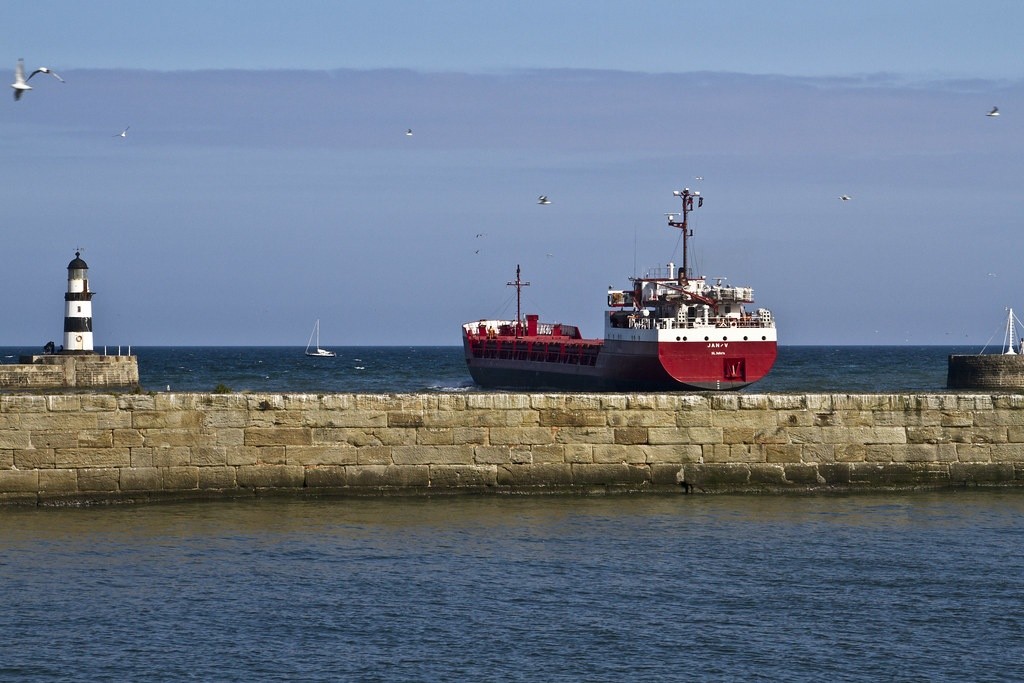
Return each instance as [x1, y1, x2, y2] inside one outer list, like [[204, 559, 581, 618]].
[[462, 188, 780, 389]]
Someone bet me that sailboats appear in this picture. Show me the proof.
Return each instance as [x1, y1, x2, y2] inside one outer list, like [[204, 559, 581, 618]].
[[304, 318, 336, 358]]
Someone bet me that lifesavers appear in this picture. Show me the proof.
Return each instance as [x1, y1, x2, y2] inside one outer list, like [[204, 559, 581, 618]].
[[730, 321, 737, 328], [76, 336, 82, 342]]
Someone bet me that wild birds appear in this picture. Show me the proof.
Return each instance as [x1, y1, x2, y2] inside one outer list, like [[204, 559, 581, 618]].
[[406, 128, 413, 137], [542, 195, 551, 206], [115, 126, 130, 138], [476, 249, 480, 255], [839, 194, 854, 201], [984, 106, 1000, 117], [476, 233, 487, 239], [11, 58, 64, 101]]
